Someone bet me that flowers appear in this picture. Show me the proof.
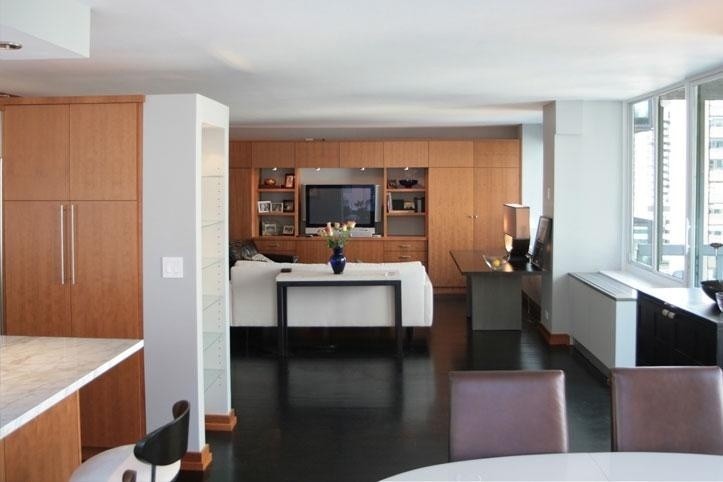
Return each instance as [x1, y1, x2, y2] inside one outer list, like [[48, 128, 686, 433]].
[[317, 221, 356, 249]]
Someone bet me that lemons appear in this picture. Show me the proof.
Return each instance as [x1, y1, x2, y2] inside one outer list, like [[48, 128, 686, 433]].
[[492, 259, 500, 266]]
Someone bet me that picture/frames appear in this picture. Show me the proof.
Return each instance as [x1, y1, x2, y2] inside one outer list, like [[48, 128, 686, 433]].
[[270, 203, 282, 213], [530, 215, 552, 271], [257, 200, 271, 213], [282, 200, 293, 212], [281, 224, 294, 235], [261, 221, 278, 236], [284, 173, 294, 189]]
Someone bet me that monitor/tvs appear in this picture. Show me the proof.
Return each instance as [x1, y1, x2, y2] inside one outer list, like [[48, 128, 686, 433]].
[[306, 184, 375, 227]]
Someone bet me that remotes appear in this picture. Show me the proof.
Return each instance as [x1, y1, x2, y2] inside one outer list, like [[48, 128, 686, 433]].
[[296, 234, 313, 237]]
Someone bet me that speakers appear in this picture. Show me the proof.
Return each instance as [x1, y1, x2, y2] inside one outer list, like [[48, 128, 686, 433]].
[[334, 232, 372, 237], [300, 185, 306, 222], [375, 185, 382, 222]]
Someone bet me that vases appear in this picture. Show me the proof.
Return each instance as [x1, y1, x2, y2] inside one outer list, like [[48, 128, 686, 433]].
[[329, 248, 346, 274]]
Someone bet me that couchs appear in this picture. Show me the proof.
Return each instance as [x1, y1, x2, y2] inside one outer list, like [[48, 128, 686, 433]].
[[227, 260, 434, 345], [228, 238, 299, 269]]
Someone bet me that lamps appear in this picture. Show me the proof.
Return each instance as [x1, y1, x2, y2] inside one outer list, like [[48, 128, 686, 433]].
[[501, 202, 531, 267]]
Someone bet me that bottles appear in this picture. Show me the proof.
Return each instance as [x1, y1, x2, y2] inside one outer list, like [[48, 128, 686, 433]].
[[415, 197, 421, 212]]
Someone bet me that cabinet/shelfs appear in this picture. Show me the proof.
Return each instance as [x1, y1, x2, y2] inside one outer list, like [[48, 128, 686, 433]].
[[383, 240, 427, 269], [383, 168, 428, 239], [297, 240, 384, 263], [633, 285, 722, 366], [568, 271, 637, 380], [426, 168, 519, 297], [228, 139, 520, 168], [258, 168, 295, 237], [256, 239, 299, 256], [229, 169, 260, 239], [0, 95, 144, 447]]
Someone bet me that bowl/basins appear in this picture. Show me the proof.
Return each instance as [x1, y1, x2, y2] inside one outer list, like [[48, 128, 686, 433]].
[[715, 292, 723, 312], [482, 254, 510, 272], [700, 280, 722, 302]]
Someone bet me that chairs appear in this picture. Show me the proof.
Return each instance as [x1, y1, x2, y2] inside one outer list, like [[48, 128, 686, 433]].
[[606, 367, 722, 458], [69, 398, 190, 482], [448, 369, 569, 460]]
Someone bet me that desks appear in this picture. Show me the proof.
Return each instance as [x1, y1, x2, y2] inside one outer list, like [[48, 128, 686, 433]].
[[276, 270, 402, 366], [0, 334, 144, 482], [448, 248, 543, 331], [377, 452, 723, 482]]
